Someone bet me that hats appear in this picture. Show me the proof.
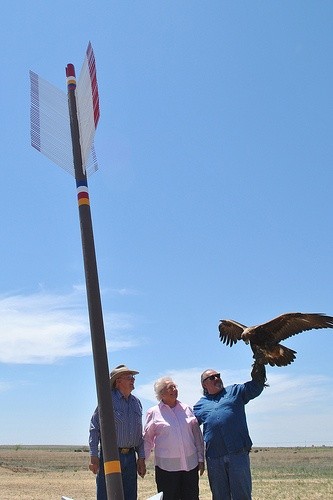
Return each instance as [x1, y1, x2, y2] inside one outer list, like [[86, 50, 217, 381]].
[[110, 365, 139, 384]]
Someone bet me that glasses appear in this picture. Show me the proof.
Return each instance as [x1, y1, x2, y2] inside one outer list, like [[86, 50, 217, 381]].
[[124, 375, 135, 380], [203, 373, 220, 383]]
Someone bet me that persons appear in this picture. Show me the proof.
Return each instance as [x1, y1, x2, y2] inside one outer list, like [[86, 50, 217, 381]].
[[89, 365, 146, 500], [192, 358, 267, 500], [142, 377, 205, 500]]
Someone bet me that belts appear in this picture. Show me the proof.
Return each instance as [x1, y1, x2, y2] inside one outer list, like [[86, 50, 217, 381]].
[[118, 448, 135, 454]]
